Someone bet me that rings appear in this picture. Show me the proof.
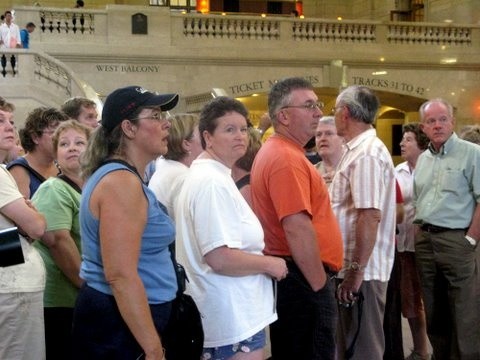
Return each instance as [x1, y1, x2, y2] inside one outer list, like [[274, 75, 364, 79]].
[[338, 284, 341, 288], [349, 292, 352, 296]]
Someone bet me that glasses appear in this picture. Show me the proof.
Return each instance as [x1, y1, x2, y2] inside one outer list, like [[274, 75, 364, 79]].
[[331, 106, 343, 114], [130, 113, 165, 121], [279, 103, 325, 110], [221, 126, 248, 136]]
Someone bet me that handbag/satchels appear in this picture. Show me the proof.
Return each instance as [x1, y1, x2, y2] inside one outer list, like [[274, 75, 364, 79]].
[[164, 294, 204, 360]]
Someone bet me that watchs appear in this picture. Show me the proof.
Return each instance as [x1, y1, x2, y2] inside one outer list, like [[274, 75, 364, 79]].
[[348, 262, 366, 271]]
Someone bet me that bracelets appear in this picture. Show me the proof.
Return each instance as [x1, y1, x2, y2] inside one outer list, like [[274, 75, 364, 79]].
[[465, 235, 476, 245]]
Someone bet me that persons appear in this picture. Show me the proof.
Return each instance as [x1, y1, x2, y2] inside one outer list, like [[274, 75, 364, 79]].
[[313, 115, 345, 191], [0, 96, 47, 360], [232, 111, 277, 211], [326, 86, 396, 360], [7, 97, 100, 360], [20, 22, 35, 49], [394, 124, 430, 360], [148, 114, 202, 221], [173, 97, 288, 360], [72, 0, 84, 34], [71, 85, 179, 360], [412, 99, 480, 360], [0, 10, 23, 77], [248, 77, 344, 360]]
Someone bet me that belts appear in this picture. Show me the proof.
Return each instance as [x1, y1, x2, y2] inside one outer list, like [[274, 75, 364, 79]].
[[419, 223, 452, 234]]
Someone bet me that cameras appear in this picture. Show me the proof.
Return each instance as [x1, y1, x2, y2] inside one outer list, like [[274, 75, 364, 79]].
[[0, 226, 25, 268]]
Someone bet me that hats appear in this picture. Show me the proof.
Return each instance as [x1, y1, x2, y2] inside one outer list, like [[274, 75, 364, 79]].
[[101, 86, 179, 132]]
